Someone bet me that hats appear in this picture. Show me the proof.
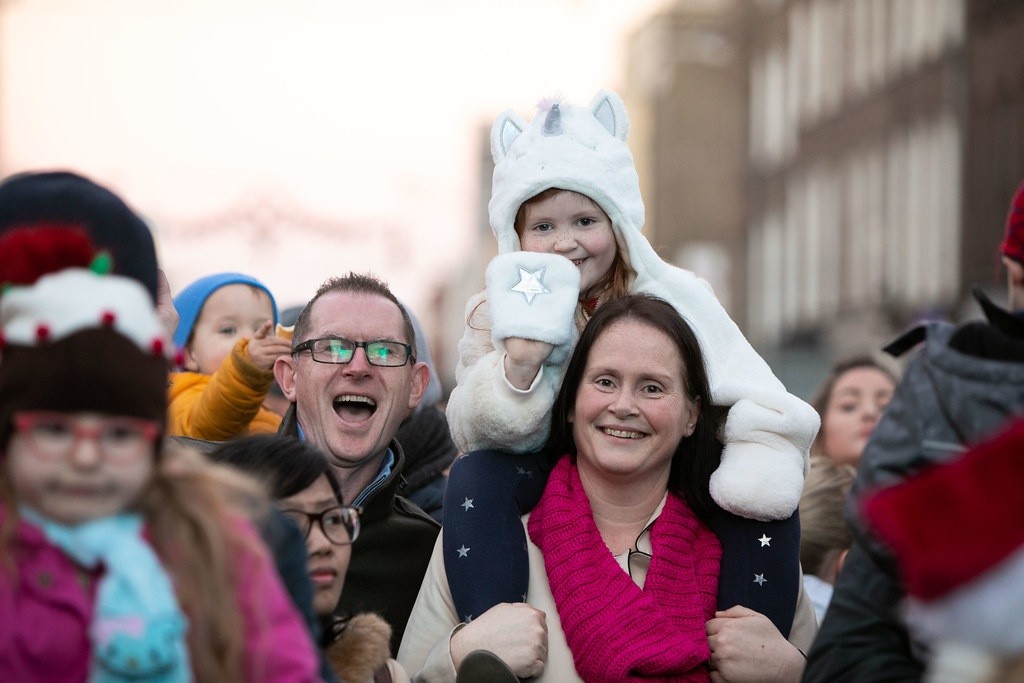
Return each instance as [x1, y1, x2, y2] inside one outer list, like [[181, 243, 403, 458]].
[[1000, 179, 1024, 263], [0, 267, 169, 458], [171, 273, 277, 348], [0, 172, 159, 304]]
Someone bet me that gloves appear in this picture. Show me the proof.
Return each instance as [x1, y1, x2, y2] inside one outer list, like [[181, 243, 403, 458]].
[[709, 439, 804, 522], [485, 251, 581, 366]]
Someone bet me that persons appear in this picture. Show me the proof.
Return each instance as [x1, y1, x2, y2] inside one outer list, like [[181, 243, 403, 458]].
[[0, 90, 1023, 683]]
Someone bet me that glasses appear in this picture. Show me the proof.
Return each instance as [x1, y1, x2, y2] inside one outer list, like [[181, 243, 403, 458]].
[[281, 505, 363, 546], [12, 412, 158, 460], [291, 336, 416, 367]]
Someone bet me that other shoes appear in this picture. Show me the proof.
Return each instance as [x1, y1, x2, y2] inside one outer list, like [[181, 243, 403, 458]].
[[456, 649, 520, 683]]
